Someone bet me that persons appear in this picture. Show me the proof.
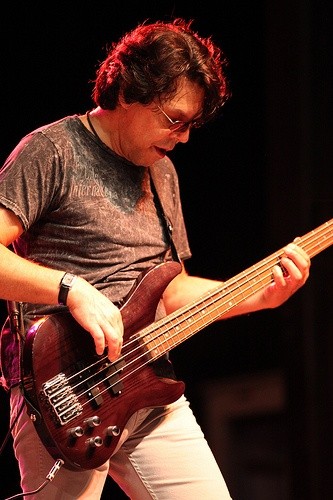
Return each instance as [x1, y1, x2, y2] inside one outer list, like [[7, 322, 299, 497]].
[[0, 25, 313, 500]]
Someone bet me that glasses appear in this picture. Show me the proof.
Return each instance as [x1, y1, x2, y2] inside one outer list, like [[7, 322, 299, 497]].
[[151, 98, 205, 132]]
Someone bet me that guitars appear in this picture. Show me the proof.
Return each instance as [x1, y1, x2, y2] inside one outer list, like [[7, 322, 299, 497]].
[[19, 214, 332, 473]]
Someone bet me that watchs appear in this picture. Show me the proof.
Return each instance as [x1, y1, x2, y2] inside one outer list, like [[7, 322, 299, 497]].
[[57, 271, 75, 304]]
